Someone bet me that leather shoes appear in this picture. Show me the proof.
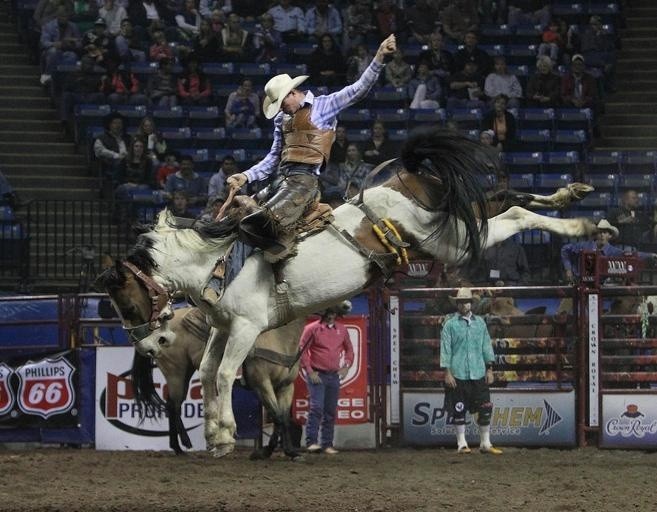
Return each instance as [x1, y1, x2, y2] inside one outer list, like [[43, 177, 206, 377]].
[[200, 288, 217, 306]]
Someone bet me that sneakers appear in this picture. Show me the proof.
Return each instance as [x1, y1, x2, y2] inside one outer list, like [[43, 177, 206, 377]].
[[306, 444, 322, 452], [322, 447, 339, 454], [479, 446, 503, 454], [458, 446, 471, 454]]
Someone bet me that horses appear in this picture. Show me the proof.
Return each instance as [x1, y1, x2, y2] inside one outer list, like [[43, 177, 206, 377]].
[[95, 122, 600, 459], [131, 299, 353, 461]]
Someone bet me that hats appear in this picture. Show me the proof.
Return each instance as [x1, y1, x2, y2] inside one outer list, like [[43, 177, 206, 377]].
[[101, 111, 129, 131], [157, 148, 181, 161], [94, 18, 107, 28], [80, 44, 102, 58], [449, 288, 481, 303], [597, 219, 619, 237], [463, 56, 483, 65], [263, 74, 309, 120], [571, 53, 585, 63]]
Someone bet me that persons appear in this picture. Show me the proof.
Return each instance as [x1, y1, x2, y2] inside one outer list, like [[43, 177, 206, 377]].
[[558, 220, 646, 320], [33, 0, 611, 227], [297, 308, 354, 454], [437, 283, 499, 454], [199, 33, 397, 303]]
